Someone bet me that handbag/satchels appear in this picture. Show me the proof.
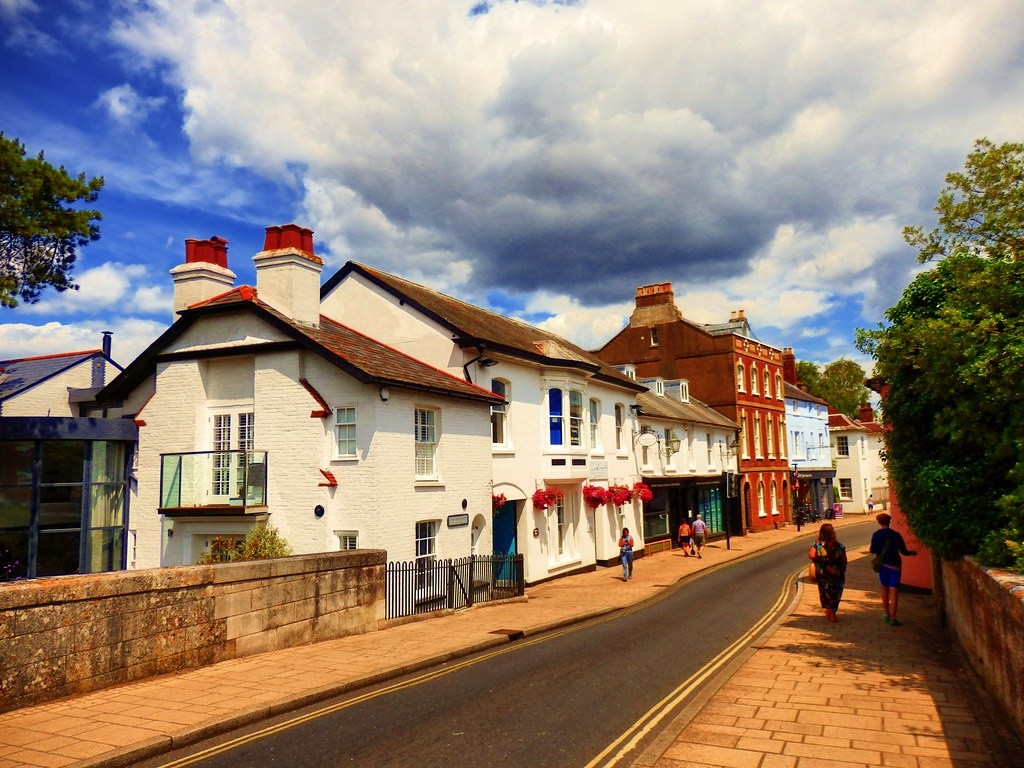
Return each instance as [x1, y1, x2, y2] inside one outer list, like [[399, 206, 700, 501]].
[[691, 543, 695, 555], [871, 555, 885, 573], [809, 562, 816, 582]]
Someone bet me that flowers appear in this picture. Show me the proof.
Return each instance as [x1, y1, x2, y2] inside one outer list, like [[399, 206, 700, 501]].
[[492, 494, 510, 517], [583, 482, 653, 509], [531, 488, 565, 511]]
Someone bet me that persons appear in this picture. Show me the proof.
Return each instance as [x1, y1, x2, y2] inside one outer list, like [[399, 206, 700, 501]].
[[870, 513, 918, 626], [678, 515, 709, 558], [809, 524, 847, 622], [866, 494, 876, 515], [619, 527, 634, 581]]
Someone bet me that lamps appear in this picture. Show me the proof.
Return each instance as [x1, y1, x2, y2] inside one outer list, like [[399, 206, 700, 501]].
[[657, 432, 681, 459], [630, 405, 643, 410], [719, 440, 740, 461], [379, 384, 390, 401], [478, 358, 498, 366]]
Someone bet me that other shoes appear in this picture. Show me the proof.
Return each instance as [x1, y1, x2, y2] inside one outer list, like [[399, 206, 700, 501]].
[[686, 550, 689, 556], [826, 612, 837, 623], [697, 551, 702, 558], [890, 619, 903, 627], [881, 612, 891, 622]]
